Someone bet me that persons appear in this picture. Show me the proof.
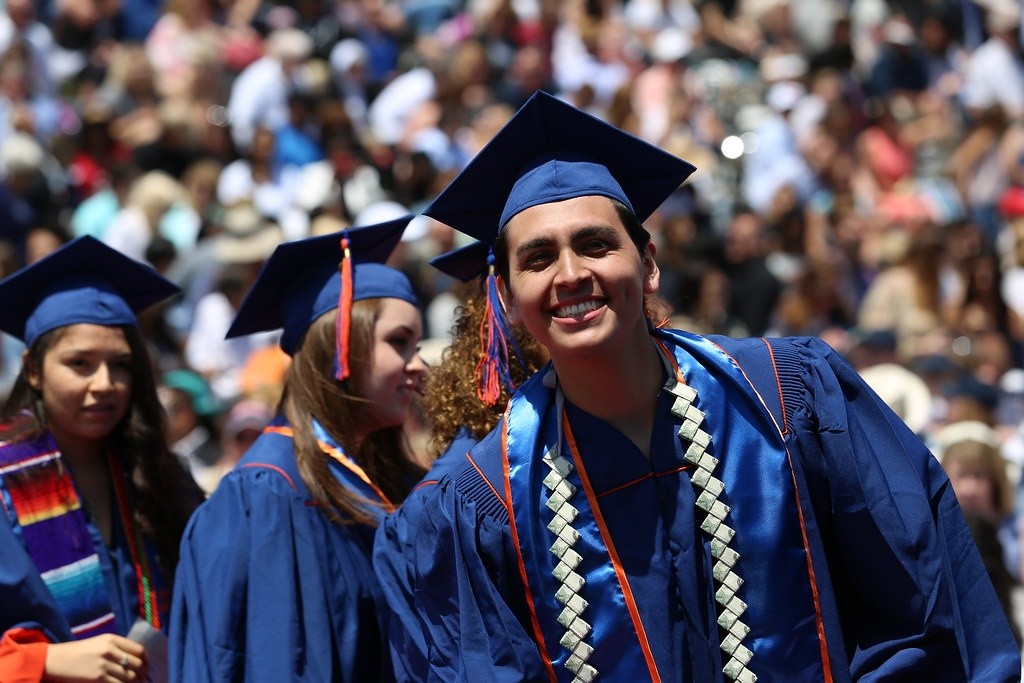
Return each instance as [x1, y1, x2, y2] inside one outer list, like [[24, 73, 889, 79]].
[[168, 214, 427, 683], [0, 234, 205, 683], [0, 0, 1023, 642], [372, 88, 1021, 683]]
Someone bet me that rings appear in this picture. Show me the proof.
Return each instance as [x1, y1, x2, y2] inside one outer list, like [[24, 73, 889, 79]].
[[121, 656, 129, 667]]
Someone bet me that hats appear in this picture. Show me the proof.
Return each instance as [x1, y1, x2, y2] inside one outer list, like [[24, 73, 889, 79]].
[[222, 213, 421, 381], [420, 88, 698, 407], [429, 241, 486, 284], [0, 233, 182, 355]]
